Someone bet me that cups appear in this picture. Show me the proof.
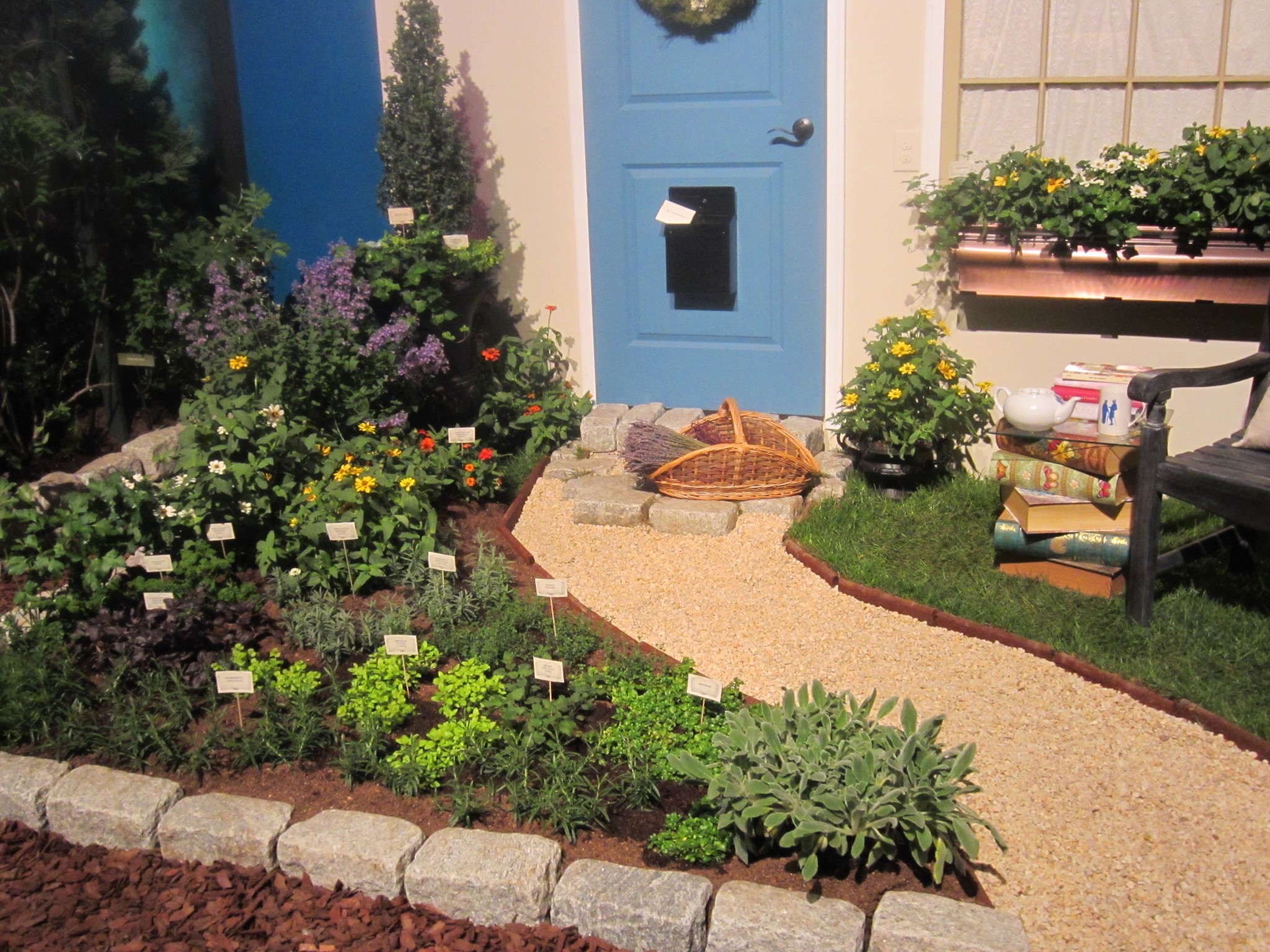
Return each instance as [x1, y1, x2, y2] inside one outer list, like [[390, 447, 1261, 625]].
[[1097, 386, 1147, 436]]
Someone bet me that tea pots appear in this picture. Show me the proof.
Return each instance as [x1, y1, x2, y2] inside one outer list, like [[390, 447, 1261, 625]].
[[993, 386, 1081, 432]]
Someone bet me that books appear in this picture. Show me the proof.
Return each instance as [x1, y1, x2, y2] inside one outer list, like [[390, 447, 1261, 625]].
[[989, 362, 1172, 598]]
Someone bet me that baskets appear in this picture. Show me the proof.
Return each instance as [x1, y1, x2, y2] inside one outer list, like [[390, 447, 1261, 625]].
[[643, 397, 821, 500]]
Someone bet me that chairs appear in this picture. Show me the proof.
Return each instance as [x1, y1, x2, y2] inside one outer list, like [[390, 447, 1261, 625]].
[[1127, 293, 1270, 633]]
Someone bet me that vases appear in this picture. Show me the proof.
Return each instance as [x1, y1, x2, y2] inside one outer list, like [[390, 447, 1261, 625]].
[[826, 427, 956, 506], [948, 218, 1270, 306]]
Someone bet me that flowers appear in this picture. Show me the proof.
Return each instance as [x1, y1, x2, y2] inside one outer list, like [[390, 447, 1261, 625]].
[[905, 128, 1270, 258], [838, 298, 993, 450]]
